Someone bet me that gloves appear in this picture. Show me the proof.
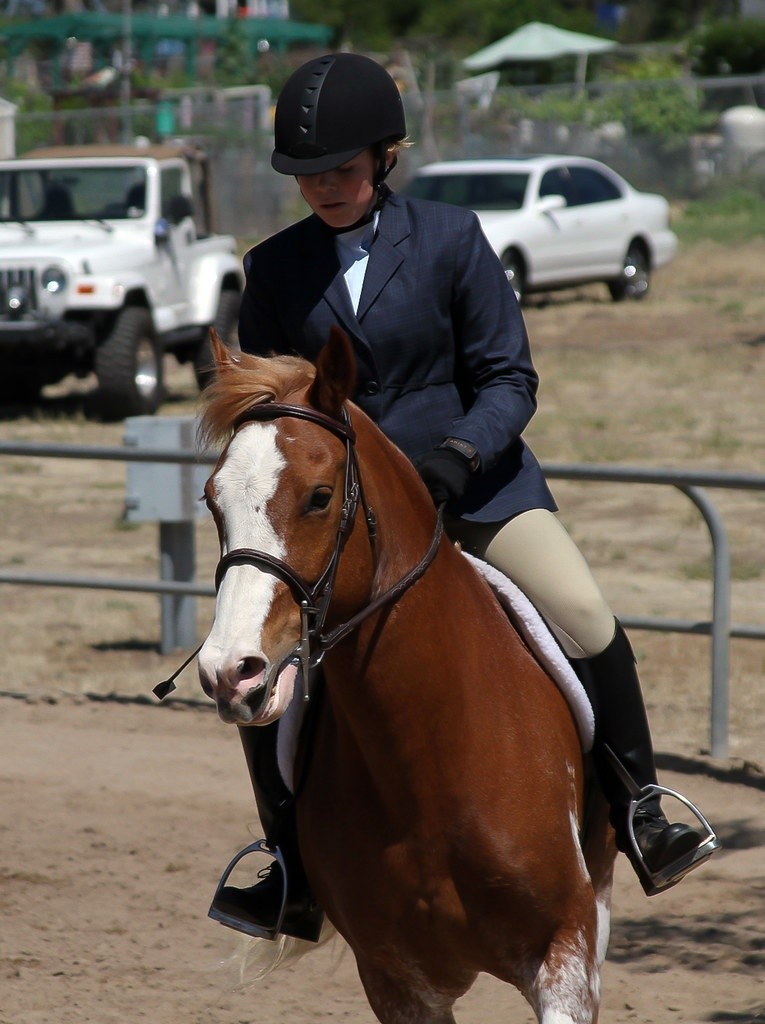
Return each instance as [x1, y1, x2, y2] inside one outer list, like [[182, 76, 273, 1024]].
[[416, 436, 481, 508]]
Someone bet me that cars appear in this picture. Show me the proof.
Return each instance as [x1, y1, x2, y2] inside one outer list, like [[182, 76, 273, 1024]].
[[405, 154, 680, 306]]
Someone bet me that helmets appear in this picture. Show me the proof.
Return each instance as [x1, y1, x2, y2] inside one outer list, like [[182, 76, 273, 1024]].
[[271, 52, 406, 175]]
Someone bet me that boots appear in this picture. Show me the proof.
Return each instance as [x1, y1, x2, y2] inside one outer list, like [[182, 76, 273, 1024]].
[[212, 717, 325, 943], [564, 615, 704, 896]]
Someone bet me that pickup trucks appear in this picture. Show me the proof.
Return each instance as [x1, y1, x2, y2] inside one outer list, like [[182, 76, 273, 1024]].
[[1, 153, 247, 420]]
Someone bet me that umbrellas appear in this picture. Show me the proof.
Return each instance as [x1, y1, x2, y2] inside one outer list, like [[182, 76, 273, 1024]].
[[467, 23, 617, 70]]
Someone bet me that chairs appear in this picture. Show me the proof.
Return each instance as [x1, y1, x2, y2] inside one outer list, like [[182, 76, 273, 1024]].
[[39, 185, 75, 220], [125, 183, 145, 209]]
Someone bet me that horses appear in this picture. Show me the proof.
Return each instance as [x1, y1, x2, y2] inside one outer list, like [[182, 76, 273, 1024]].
[[196, 322, 620, 1024]]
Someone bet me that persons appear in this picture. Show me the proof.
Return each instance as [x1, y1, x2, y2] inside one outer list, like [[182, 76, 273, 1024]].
[[217, 52, 704, 944]]
